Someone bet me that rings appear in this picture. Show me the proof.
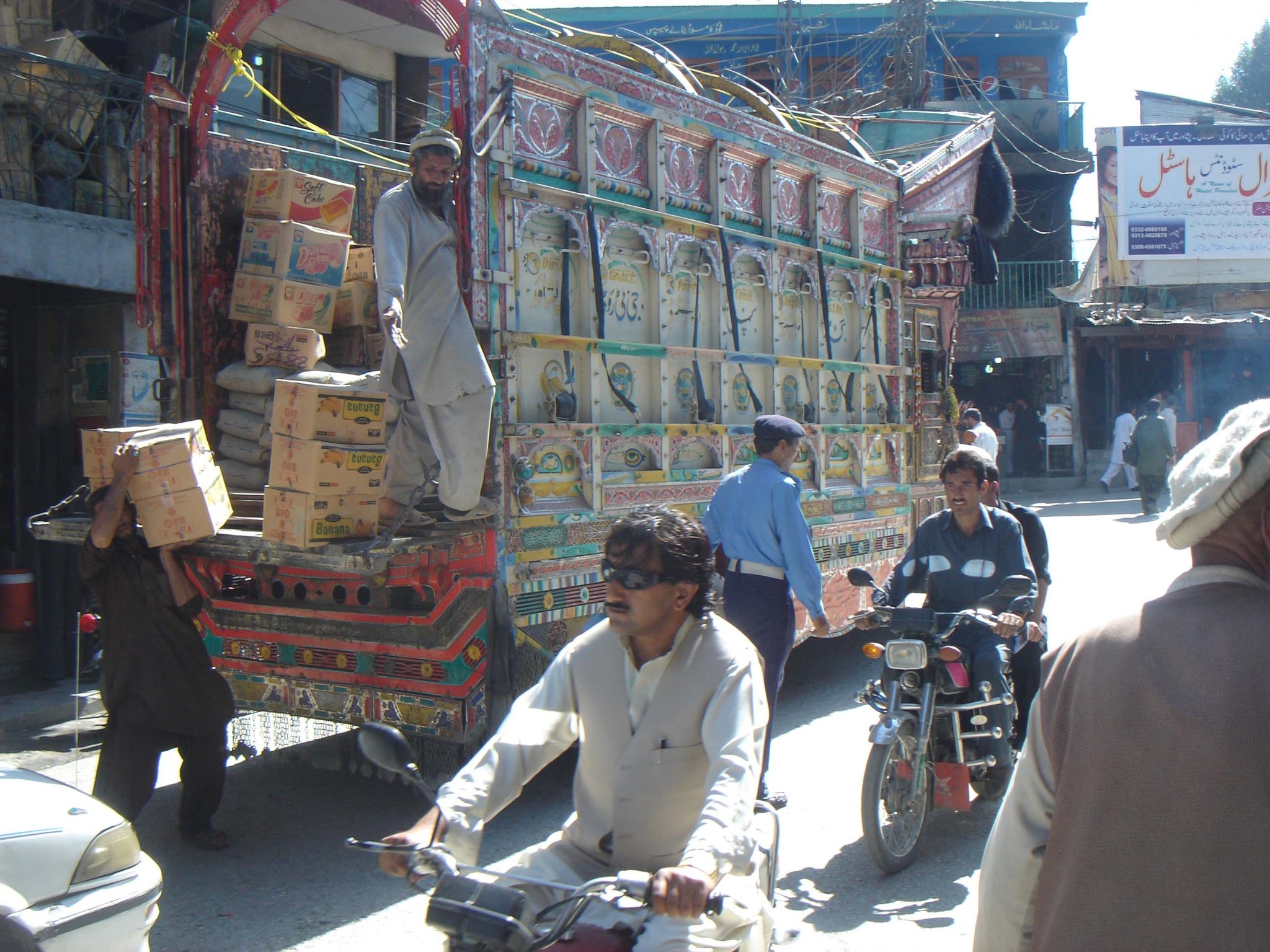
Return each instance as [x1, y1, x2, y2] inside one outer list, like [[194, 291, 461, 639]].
[[133, 451, 139, 457]]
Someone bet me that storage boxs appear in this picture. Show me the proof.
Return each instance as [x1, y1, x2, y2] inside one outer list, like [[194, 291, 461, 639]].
[[138, 466, 235, 549], [271, 379, 388, 445], [121, 417, 213, 501], [269, 432, 386, 496], [0, 7, 150, 221], [226, 163, 392, 372], [80, 425, 157, 479], [262, 483, 378, 551]]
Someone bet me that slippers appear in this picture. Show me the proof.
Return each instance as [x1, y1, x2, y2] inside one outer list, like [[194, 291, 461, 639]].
[[444, 495, 501, 521], [378, 507, 437, 526]]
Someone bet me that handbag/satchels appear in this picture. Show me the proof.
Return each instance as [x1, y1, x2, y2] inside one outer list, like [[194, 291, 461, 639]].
[[1122, 440, 1139, 467]]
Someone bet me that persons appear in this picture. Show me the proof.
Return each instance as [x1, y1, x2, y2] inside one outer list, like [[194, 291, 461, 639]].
[[968, 392, 1269, 952], [370, 504, 780, 951], [972, 452, 1050, 749], [704, 412, 835, 813], [374, 127, 504, 529], [961, 389, 1184, 512], [849, 446, 1043, 793], [75, 439, 237, 852], [1097, 145, 1144, 285]]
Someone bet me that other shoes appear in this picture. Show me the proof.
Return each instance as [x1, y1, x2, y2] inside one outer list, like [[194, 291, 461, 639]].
[[1132, 485, 1141, 491], [1100, 480, 1109, 493], [179, 828, 231, 851], [985, 765, 1012, 802], [1144, 499, 1160, 515]]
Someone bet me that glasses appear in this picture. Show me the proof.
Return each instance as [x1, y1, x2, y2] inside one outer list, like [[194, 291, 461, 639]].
[[601, 558, 677, 591]]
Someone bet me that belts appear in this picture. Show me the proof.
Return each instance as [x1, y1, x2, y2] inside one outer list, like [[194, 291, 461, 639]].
[[727, 558, 784, 580]]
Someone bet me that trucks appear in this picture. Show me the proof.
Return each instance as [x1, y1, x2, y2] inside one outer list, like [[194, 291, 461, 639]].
[[28, 0, 995, 755]]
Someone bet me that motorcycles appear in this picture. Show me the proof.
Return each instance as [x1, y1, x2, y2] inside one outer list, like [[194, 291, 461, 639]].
[[346, 720, 787, 952], [846, 565, 1032, 875]]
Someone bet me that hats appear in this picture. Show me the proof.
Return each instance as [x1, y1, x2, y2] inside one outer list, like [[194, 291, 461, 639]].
[[409, 128, 463, 159], [753, 414, 806, 439], [1155, 398, 1270, 550]]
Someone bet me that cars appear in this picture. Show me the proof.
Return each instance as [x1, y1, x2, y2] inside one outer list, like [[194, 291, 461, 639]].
[[0, 757, 164, 952]]
